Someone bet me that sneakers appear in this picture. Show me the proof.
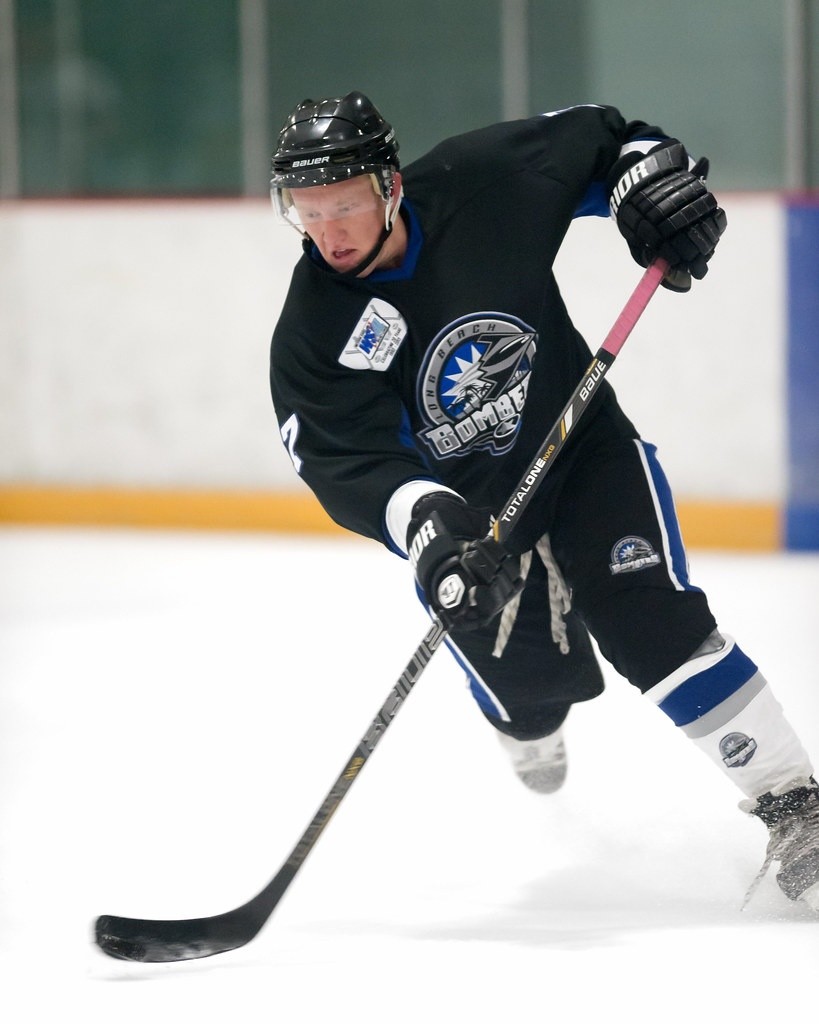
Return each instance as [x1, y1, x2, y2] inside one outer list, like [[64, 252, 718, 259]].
[[495, 727, 566, 794], [738, 770, 819, 916]]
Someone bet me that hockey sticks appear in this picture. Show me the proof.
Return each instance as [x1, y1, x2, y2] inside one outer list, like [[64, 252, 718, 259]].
[[92, 153, 712, 966]]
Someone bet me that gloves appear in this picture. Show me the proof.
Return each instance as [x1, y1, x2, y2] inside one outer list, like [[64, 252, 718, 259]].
[[406, 491, 526, 635], [604, 138, 728, 293]]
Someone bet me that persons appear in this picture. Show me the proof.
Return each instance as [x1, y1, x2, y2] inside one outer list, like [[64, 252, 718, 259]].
[[268, 90, 819, 918]]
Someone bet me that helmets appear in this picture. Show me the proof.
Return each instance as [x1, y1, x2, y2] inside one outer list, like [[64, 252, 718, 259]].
[[268, 91, 403, 239]]
